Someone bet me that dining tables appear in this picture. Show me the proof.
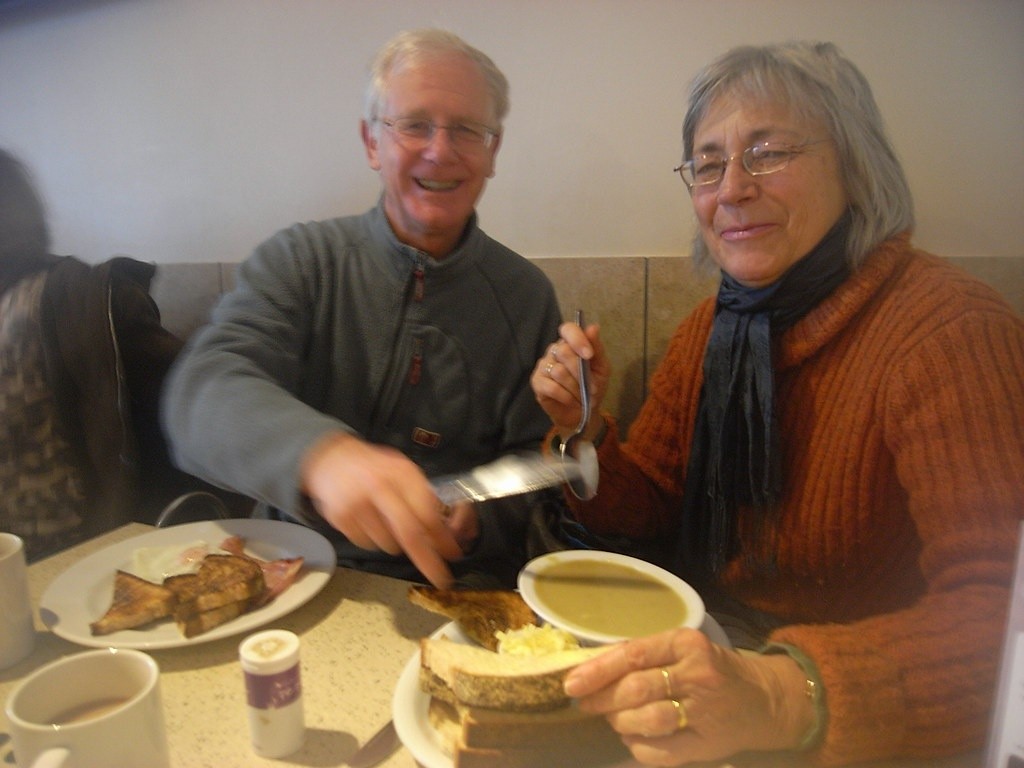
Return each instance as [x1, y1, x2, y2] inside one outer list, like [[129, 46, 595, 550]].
[[0, 522, 456, 768]]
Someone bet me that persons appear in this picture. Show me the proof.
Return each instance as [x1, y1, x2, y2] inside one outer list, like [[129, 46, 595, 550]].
[[531, 41, 1024, 768], [161, 27, 563, 590], [0, 148, 257, 564]]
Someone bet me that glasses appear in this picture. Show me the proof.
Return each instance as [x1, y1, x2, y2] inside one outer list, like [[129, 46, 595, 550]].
[[672, 139, 833, 186], [373, 116, 499, 151]]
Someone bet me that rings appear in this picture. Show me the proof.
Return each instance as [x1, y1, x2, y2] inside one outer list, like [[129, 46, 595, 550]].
[[551, 343, 559, 355], [660, 665, 673, 697], [671, 699, 687, 728], [546, 361, 557, 375]]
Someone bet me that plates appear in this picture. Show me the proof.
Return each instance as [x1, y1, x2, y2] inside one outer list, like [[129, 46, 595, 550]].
[[39, 519, 338, 649], [395, 610, 733, 768]]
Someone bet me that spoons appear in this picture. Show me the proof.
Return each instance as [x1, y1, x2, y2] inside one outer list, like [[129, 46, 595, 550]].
[[562, 310, 599, 499]]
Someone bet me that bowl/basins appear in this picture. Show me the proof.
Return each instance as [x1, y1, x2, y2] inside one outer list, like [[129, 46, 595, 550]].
[[517, 550, 706, 648]]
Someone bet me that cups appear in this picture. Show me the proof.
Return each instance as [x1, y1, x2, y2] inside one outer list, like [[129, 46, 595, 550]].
[[4, 648, 171, 768], [0, 532, 38, 671]]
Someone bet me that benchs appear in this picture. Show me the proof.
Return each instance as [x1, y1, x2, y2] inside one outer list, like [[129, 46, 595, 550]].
[[147, 256, 1024, 447]]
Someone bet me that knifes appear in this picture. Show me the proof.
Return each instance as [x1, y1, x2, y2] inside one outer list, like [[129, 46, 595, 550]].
[[346, 720, 402, 768], [423, 453, 583, 506]]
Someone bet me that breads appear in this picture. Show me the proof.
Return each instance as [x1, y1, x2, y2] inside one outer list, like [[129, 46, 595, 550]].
[[408, 581, 618, 768], [88, 552, 307, 640]]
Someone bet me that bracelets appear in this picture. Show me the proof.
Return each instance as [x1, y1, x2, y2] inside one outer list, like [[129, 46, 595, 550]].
[[804, 676, 817, 705]]
